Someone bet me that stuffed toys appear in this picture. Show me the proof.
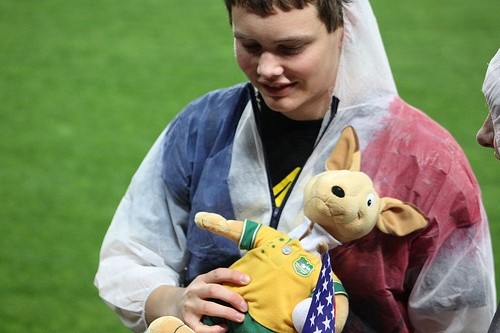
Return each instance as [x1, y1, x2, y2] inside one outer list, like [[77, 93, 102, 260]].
[[144, 126, 430, 333]]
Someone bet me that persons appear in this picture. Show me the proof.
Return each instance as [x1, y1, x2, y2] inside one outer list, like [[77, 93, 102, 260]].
[[94, 0, 498, 333]]
